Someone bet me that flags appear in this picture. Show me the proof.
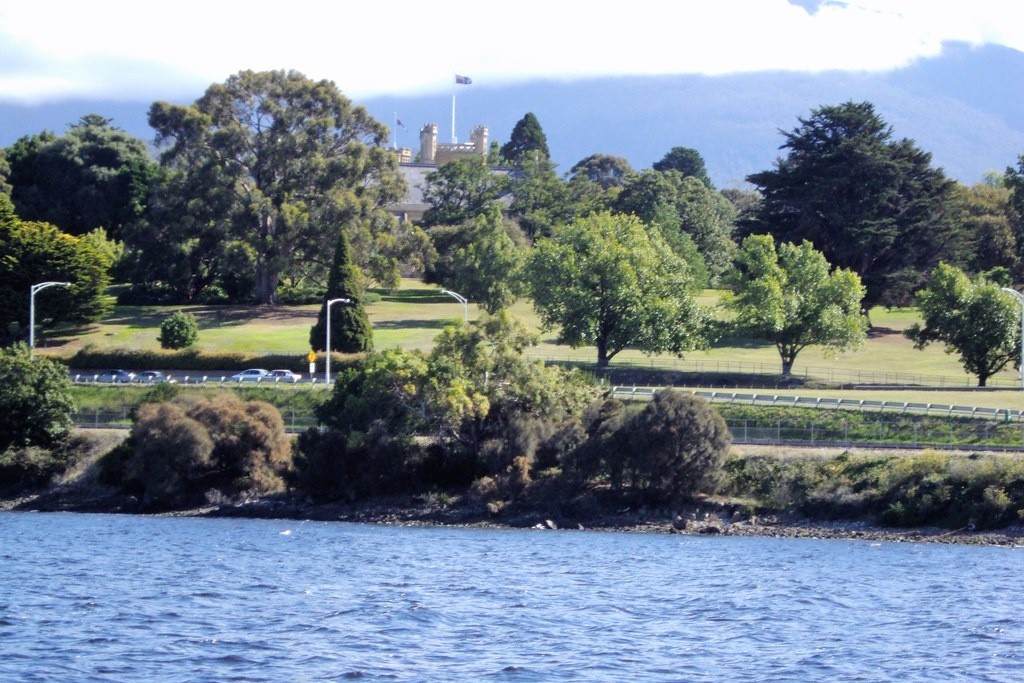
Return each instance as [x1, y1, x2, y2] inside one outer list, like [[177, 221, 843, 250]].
[[396, 116, 407, 131], [456, 76, 472, 84]]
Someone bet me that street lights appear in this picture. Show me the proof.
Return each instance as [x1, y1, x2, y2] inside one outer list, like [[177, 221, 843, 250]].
[[441, 289, 468, 323], [29, 281, 71, 358], [325, 299, 350, 386]]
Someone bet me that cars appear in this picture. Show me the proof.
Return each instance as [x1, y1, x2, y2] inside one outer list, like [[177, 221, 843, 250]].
[[98, 371, 136, 382], [263, 371, 303, 381], [231, 369, 276, 382], [136, 371, 169, 383]]
